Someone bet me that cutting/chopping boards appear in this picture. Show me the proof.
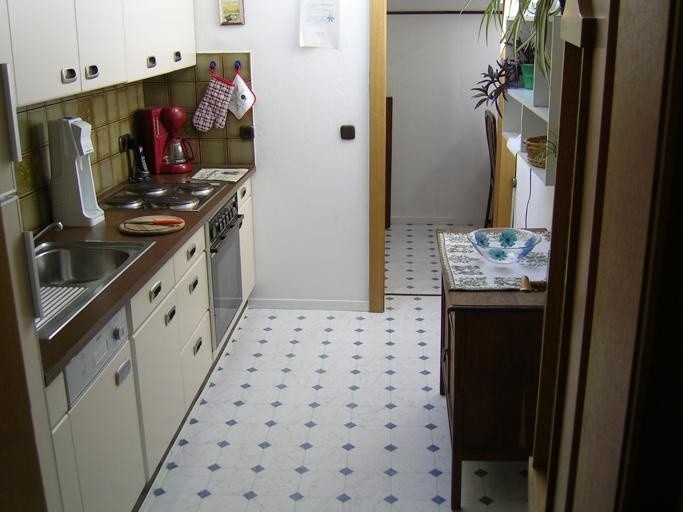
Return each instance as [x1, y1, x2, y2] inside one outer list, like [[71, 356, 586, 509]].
[[118, 214, 185, 237]]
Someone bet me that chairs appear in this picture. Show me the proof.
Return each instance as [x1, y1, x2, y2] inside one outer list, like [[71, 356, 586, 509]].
[[485, 109, 496, 227]]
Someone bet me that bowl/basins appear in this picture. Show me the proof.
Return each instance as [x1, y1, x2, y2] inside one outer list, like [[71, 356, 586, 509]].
[[466, 227, 541, 265]]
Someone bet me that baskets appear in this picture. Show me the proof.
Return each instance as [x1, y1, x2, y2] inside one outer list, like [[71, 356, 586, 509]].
[[526, 135, 547, 169]]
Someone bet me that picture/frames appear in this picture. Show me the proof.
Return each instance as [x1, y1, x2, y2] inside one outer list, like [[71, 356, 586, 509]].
[[218, 0, 246, 25]]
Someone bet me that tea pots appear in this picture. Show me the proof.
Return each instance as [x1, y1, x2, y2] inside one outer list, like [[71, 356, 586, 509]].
[[160, 135, 195, 166]]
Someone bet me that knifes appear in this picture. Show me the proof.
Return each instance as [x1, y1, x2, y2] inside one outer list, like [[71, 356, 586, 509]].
[[118, 219, 183, 225]]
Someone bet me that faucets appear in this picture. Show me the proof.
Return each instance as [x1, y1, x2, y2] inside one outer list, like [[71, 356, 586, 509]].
[[32, 220, 64, 243]]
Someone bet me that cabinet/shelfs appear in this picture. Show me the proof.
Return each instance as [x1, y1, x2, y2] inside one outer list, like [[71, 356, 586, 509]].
[[66, 337, 148, 511], [128, 253, 184, 483], [0, 2, 196, 108], [237, 177, 256, 300], [501, 1, 564, 186], [174, 227, 211, 427], [437, 228, 551, 511]]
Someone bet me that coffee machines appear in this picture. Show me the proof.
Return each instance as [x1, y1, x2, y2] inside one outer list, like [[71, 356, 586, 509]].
[[132, 105, 195, 175]]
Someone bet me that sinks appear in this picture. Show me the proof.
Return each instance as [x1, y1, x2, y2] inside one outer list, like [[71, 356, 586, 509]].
[[34, 241, 135, 287]]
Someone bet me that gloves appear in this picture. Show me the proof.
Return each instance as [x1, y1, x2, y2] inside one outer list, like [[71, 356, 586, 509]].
[[192, 76, 234, 132]]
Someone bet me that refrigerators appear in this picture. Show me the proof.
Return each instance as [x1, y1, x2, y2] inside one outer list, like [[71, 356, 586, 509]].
[[0, 63, 64, 511]]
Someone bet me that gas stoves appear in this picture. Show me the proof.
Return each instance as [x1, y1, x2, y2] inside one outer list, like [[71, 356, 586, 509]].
[[98, 180, 228, 212]]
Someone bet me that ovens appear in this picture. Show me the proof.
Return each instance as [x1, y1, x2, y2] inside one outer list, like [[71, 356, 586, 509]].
[[208, 192, 244, 351]]
